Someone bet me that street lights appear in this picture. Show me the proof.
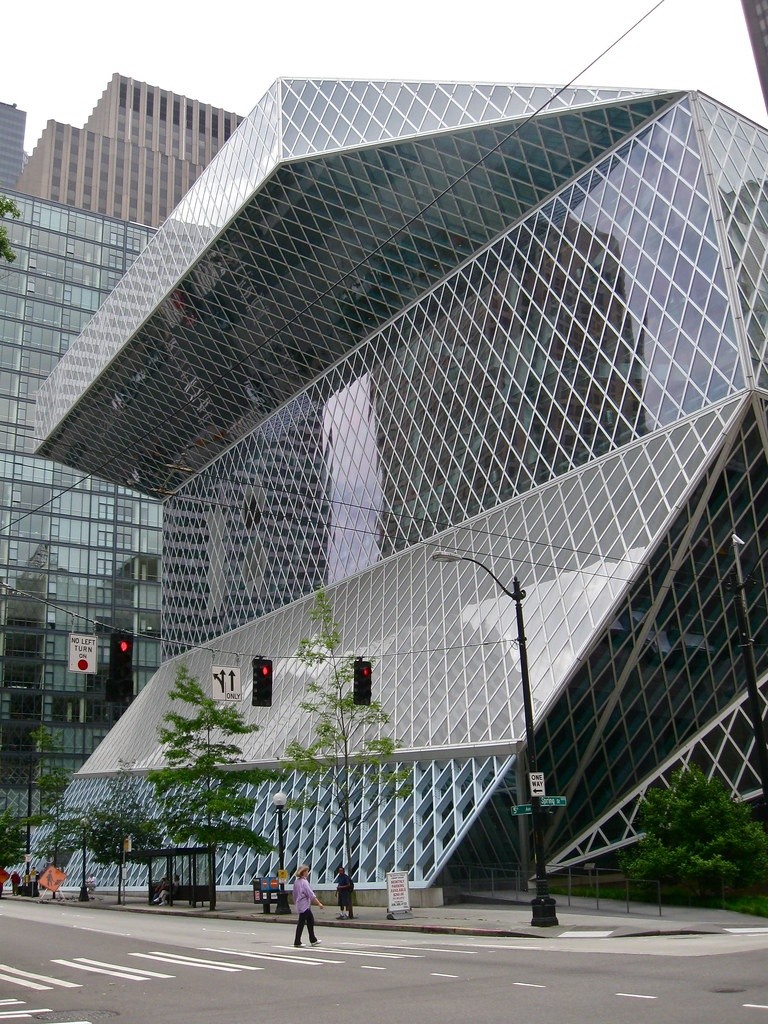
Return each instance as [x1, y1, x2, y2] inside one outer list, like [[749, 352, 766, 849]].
[[272, 792, 293, 915], [431, 550, 559, 928], [78, 818, 93, 902]]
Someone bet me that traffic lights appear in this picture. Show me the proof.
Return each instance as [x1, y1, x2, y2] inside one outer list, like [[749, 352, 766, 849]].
[[106, 632, 134, 703], [353, 660, 373, 706], [251, 658, 273, 708]]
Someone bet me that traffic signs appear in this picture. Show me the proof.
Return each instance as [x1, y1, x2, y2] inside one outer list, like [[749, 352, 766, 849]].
[[528, 771, 547, 797]]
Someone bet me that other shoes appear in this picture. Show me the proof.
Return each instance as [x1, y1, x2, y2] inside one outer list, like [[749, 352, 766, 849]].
[[159, 902, 166, 906], [154, 898, 159, 902], [336, 914, 349, 919], [311, 940, 322, 946], [294, 944, 306, 947]]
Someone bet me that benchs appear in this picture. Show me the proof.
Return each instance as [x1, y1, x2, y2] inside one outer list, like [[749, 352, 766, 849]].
[[166, 886, 209, 908]]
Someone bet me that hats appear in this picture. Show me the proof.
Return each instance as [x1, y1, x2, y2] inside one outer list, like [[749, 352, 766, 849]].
[[296, 864, 309, 877]]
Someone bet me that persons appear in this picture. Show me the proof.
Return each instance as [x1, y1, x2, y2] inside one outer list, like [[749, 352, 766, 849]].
[[335, 867, 353, 920], [151, 874, 180, 906], [10, 871, 20, 896], [30, 866, 39, 880], [292, 865, 324, 947], [85, 874, 96, 894]]
